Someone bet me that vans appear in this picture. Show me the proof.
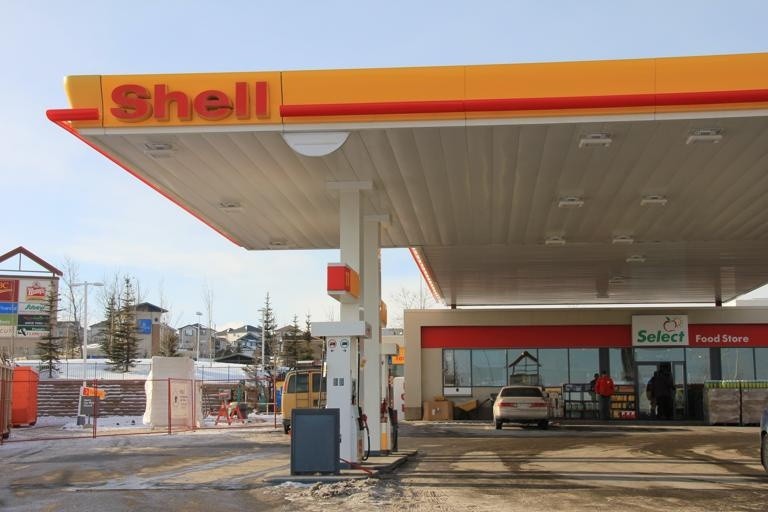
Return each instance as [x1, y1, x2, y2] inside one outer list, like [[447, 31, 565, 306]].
[[280, 360, 326, 435]]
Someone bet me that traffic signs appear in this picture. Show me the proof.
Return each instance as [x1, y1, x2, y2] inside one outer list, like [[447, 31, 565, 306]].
[[81, 388, 107, 399]]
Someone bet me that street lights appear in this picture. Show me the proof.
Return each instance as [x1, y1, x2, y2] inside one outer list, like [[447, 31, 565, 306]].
[[67, 282, 103, 363], [257, 309, 264, 373], [195, 311, 202, 365]]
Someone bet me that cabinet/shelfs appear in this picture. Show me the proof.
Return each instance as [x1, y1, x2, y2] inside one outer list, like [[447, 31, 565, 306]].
[[563, 383, 599, 419], [608, 385, 637, 420]]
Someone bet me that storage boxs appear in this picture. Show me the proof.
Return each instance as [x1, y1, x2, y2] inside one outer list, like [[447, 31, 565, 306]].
[[423, 400, 454, 421]]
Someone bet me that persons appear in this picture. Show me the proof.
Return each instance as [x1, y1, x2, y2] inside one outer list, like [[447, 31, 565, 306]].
[[589, 369, 677, 421]]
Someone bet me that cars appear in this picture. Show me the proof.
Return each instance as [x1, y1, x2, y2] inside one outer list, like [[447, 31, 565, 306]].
[[759, 408, 768, 474], [489, 386, 548, 430]]
